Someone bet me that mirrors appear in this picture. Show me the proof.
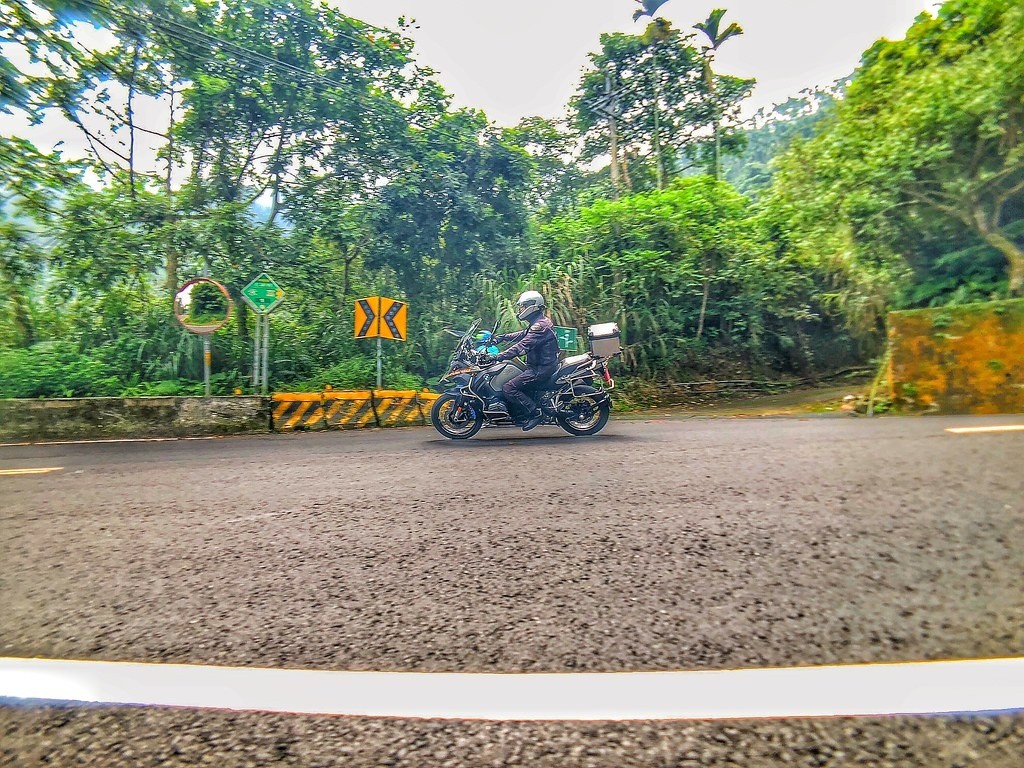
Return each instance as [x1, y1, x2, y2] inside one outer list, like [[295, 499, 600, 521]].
[[175, 281, 229, 332]]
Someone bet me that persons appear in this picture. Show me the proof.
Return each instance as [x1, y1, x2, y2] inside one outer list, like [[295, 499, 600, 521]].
[[479, 291, 560, 432]]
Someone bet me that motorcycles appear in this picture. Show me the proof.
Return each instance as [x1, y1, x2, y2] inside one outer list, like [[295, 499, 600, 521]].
[[431, 318, 623, 440]]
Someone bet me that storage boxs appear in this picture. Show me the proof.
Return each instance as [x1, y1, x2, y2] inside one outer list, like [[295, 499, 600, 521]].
[[588, 322, 620, 358]]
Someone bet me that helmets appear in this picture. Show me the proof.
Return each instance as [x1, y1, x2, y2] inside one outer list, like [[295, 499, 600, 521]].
[[515, 290, 548, 320]]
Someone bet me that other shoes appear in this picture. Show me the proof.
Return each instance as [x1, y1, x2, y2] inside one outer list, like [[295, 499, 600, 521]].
[[521, 406, 546, 432]]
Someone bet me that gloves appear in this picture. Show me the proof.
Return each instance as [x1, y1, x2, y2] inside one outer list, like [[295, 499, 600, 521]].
[[479, 353, 494, 367], [490, 335, 502, 345]]
